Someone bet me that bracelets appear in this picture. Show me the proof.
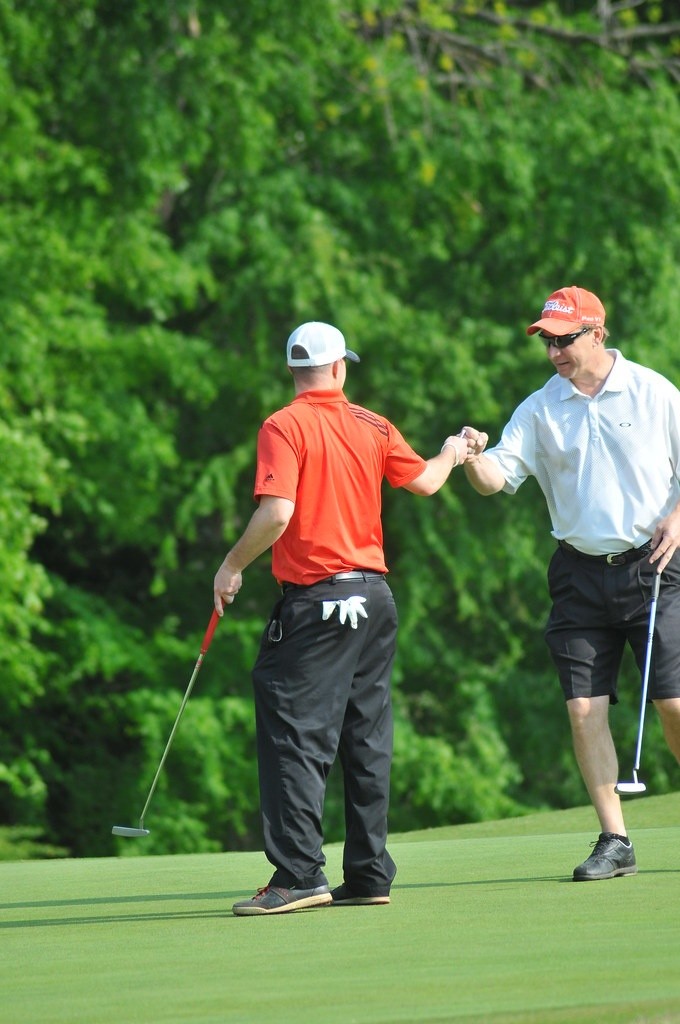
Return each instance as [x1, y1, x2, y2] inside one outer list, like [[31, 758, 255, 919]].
[[440, 443, 459, 468]]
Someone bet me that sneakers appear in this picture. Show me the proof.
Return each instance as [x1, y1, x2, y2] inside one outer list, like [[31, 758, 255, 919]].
[[573, 833, 637, 882], [232, 885, 332, 916], [330, 882, 390, 905]]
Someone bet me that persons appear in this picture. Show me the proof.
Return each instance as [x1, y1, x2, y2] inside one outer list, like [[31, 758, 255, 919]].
[[453, 285, 680, 878], [214, 321, 477, 914]]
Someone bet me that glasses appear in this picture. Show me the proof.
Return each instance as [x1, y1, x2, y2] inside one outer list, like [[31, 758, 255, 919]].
[[538, 328, 589, 349]]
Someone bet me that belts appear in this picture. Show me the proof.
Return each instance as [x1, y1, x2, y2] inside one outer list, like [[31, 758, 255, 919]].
[[558, 538, 652, 568], [325, 571, 383, 580]]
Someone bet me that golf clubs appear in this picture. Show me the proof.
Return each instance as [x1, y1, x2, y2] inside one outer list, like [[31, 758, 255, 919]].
[[111, 596, 226, 838], [613, 547, 663, 795]]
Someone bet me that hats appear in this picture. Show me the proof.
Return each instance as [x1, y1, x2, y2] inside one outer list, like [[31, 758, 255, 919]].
[[526, 286, 605, 336], [286, 322, 361, 367]]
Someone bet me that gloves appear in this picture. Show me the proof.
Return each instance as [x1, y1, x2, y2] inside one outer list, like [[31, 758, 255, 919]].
[[321, 596, 368, 629]]
